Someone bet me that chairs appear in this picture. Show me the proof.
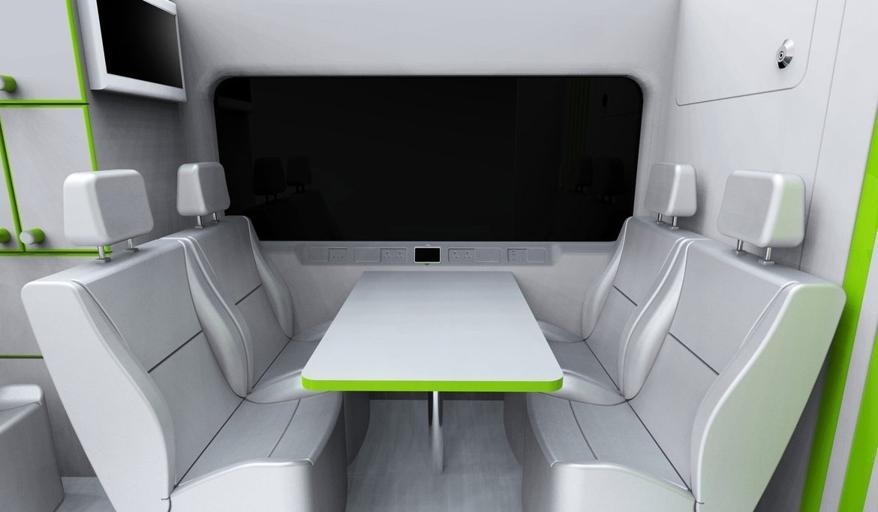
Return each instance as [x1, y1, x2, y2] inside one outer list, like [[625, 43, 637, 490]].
[[507, 165, 846, 511], [152, 161, 373, 470], [504, 161, 707, 467], [16, 165, 353, 510]]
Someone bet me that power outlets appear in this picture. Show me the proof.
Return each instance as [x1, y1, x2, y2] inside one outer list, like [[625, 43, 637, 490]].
[[379, 247, 407, 267], [450, 248, 475, 265], [328, 247, 347, 265]]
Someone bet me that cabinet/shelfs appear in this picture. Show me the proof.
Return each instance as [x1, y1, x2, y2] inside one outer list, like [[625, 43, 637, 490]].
[[0, 249, 133, 479], [0, 102, 185, 253], [0, 0, 177, 105]]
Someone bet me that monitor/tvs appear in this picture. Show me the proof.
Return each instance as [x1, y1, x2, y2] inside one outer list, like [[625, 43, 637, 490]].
[[78, 0, 188, 102]]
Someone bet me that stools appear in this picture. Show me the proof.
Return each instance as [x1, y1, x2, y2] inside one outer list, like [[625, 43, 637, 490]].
[[0, 383, 66, 512]]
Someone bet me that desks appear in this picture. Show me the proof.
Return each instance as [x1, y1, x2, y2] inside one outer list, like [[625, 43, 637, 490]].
[[300, 271, 566, 474]]
